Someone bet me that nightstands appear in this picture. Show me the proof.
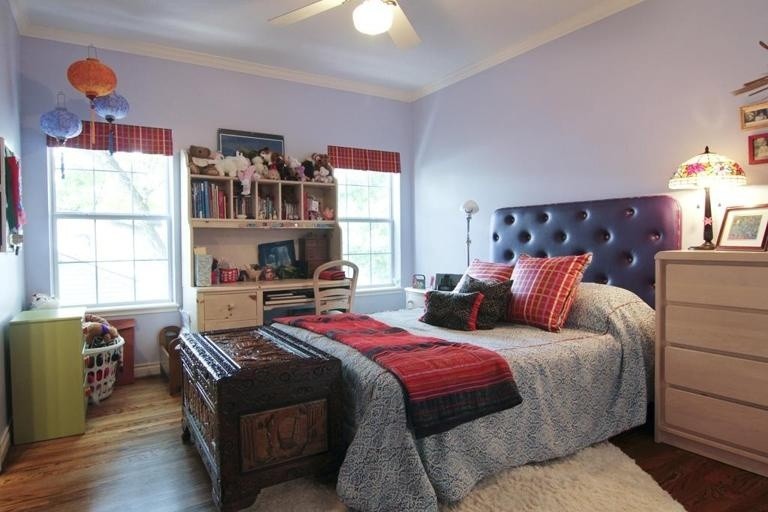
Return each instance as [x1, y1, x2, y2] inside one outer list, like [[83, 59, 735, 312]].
[[403, 287, 433, 309]]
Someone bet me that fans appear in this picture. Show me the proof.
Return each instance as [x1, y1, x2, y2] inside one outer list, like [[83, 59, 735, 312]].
[[267, 0, 421, 51]]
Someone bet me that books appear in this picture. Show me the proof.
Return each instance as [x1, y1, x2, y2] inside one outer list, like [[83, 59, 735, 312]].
[[282, 198, 299, 220], [233, 192, 253, 219], [258, 196, 275, 220], [192, 180, 228, 218]]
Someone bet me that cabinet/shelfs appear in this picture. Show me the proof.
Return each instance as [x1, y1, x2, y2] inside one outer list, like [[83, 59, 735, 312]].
[[653, 249, 768, 478], [6, 307, 87, 447], [179, 151, 342, 333]]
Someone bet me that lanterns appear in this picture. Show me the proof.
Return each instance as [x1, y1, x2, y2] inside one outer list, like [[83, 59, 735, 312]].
[[66, 46, 117, 145], [92, 90, 129, 156], [40, 93, 83, 178]]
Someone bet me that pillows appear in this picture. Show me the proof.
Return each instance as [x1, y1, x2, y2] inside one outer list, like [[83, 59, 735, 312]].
[[505, 252, 593, 333], [458, 274, 513, 329], [451, 258, 514, 293], [417, 290, 484, 330]]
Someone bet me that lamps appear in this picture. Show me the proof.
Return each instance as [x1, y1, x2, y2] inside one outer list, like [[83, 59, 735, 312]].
[[459, 200, 480, 269], [668, 146, 746, 251], [351, 0, 394, 36]]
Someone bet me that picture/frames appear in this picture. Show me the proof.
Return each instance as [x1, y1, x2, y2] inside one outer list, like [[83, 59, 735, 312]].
[[739, 102, 768, 132], [714, 204, 767, 252], [0, 138, 15, 252], [747, 132, 768, 164], [216, 128, 285, 160]]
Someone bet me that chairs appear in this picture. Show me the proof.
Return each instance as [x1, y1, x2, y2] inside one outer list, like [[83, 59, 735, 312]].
[[313, 259, 359, 315]]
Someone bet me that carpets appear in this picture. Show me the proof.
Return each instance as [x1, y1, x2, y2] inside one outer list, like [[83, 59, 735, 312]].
[[242, 439, 686, 512]]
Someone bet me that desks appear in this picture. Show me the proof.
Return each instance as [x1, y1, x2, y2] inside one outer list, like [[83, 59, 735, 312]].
[[179, 325, 342, 511]]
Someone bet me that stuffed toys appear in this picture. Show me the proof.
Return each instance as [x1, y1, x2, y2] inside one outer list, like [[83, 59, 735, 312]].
[[30, 293, 118, 346], [189, 143, 334, 195]]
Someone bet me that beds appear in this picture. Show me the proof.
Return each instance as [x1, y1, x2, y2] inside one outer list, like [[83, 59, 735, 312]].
[[269, 196, 682, 511]]
